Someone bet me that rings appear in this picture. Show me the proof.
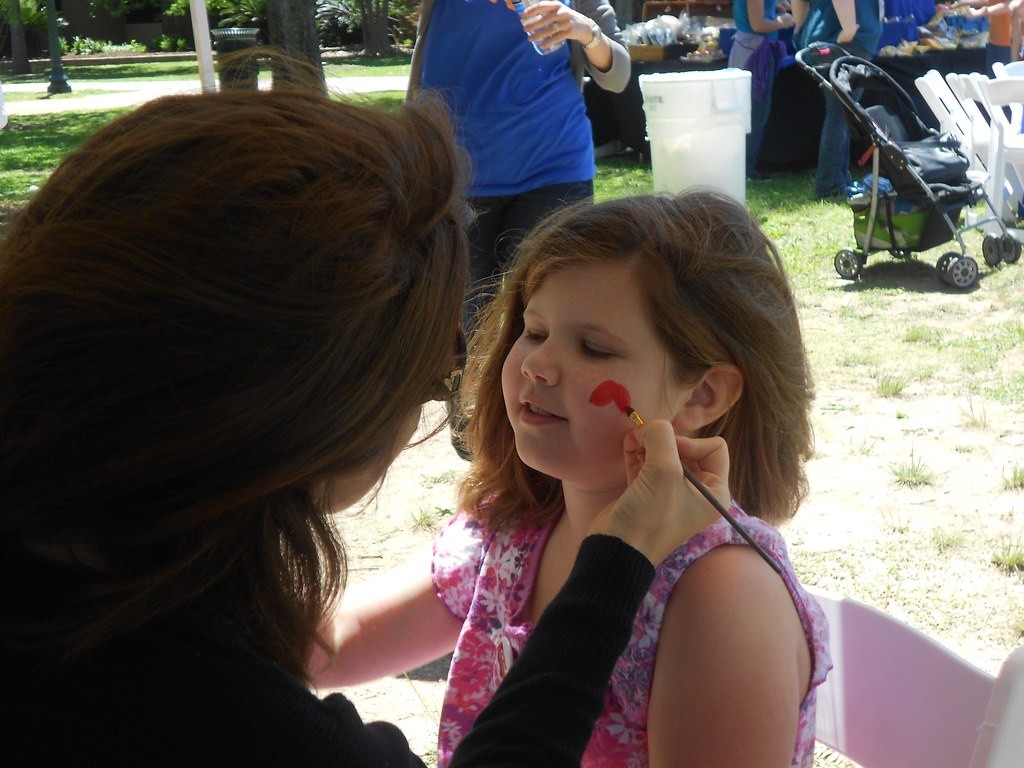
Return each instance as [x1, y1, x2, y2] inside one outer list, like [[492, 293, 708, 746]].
[[552, 22, 560, 32]]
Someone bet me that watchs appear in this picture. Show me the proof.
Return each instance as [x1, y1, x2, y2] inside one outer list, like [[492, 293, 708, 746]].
[[584, 24, 601, 49]]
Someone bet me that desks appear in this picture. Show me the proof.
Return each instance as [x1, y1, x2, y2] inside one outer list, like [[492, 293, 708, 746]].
[[864, 42, 1011, 164], [589, 58, 731, 162]]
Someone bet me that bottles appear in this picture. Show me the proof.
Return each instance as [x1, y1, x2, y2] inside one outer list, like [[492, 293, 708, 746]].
[[511, 0, 567, 56]]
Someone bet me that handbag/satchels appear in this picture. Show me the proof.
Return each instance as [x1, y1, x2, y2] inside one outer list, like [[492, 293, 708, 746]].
[[896, 133, 970, 183]]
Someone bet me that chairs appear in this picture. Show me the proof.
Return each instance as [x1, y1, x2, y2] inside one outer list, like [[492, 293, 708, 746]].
[[915, 60, 1024, 243]]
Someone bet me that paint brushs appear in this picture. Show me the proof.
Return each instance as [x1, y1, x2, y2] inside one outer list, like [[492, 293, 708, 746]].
[[623, 403, 785, 577]]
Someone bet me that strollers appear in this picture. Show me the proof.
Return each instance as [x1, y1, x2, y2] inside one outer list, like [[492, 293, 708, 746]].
[[795, 38, 1022, 291]]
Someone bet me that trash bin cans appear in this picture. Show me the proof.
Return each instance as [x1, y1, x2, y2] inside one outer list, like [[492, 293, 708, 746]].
[[638, 69, 752, 208]]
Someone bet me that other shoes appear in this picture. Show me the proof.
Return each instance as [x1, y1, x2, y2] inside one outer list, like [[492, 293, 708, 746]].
[[746, 174, 772, 184], [836, 186, 863, 197]]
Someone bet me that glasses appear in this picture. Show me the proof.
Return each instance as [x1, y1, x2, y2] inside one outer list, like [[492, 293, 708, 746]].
[[412, 327, 467, 406]]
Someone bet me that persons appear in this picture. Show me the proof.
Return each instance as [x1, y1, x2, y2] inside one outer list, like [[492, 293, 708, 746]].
[[728, 0, 796, 184], [0, 43, 732, 768], [791, 0, 882, 196], [405, 0, 630, 465], [304, 183, 835, 768], [959, 0, 1024, 79]]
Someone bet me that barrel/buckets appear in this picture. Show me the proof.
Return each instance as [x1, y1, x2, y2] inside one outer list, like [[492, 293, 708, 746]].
[[639, 72, 751, 206]]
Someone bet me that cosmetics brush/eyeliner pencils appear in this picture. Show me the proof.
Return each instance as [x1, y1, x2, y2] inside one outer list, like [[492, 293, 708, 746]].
[[624, 405, 782, 573]]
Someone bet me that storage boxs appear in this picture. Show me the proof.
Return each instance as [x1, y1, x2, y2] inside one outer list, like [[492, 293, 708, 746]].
[[628, 44, 666, 62], [667, 44, 699, 62]]
